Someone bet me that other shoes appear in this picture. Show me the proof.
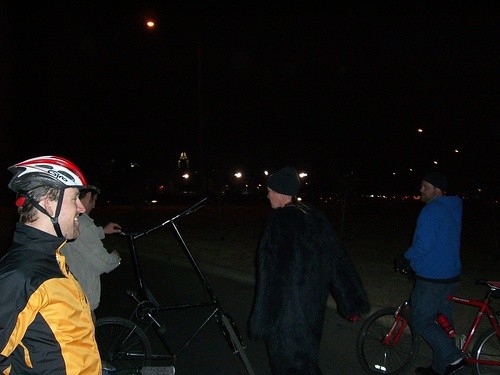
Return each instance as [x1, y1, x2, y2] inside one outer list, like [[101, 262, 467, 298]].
[[444, 359, 465, 374]]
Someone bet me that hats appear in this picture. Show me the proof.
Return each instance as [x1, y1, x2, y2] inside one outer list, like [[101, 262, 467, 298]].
[[424, 174, 450, 191], [267, 167, 301, 197]]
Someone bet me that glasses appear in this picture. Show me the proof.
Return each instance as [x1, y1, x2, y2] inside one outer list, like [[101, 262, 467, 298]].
[[8, 156, 87, 191]]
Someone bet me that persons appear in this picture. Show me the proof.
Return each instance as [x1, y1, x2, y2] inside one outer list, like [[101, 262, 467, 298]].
[[0, 155, 103, 375], [60, 178, 122, 372], [247, 166, 369, 375], [394, 172, 470, 375]]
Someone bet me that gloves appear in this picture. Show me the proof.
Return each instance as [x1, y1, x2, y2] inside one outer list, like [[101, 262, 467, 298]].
[[394, 256, 406, 270]]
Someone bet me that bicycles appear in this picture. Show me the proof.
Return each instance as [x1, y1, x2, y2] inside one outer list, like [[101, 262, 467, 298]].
[[356, 266, 500, 375], [95, 196, 257, 375]]
